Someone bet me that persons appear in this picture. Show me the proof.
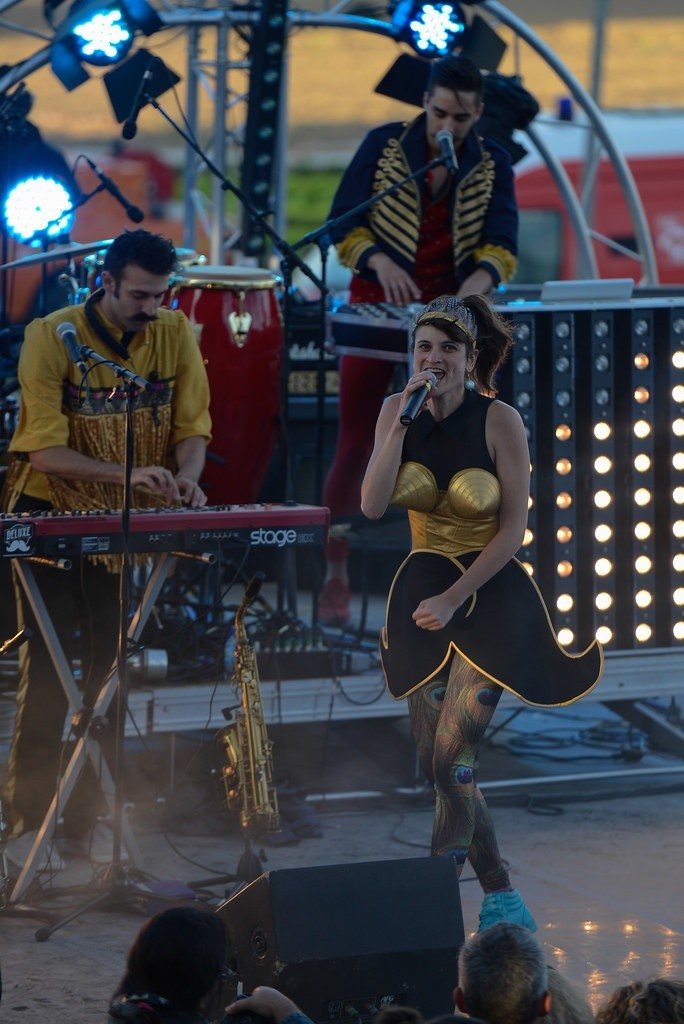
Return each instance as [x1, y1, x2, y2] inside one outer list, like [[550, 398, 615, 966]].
[[106, 909, 684, 1024], [317, 55, 519, 629], [0, 227, 213, 870], [361, 294, 605, 933]]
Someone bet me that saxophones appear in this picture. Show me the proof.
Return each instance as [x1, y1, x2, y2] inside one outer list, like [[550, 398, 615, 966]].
[[211, 570, 284, 840]]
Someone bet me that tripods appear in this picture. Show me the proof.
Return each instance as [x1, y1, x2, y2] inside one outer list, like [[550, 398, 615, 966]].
[[35, 346, 219, 944]]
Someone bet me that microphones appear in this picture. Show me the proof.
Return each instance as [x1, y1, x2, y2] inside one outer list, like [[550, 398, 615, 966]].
[[56, 322, 88, 375], [122, 63, 155, 140], [86, 158, 144, 224], [435, 130, 458, 176], [400, 371, 437, 426]]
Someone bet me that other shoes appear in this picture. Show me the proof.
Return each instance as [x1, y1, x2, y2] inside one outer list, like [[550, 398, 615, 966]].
[[321, 581, 351, 622]]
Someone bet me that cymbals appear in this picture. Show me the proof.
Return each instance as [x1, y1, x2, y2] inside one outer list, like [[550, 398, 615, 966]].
[[0, 238, 115, 272]]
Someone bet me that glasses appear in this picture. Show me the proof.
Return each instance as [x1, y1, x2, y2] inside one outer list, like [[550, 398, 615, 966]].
[[218, 956, 238, 977]]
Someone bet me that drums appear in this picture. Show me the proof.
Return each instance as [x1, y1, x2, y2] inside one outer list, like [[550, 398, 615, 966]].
[[86, 246, 203, 296], [167, 265, 286, 503]]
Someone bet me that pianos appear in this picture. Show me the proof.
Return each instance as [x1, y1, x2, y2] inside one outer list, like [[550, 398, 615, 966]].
[[1, 501, 330, 907]]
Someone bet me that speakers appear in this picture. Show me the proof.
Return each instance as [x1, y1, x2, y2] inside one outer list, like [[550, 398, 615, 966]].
[[211, 855, 466, 1024]]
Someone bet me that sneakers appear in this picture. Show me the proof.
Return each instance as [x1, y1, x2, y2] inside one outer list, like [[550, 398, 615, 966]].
[[2, 827, 65, 872], [54, 815, 127, 864], [475, 887, 537, 939]]
